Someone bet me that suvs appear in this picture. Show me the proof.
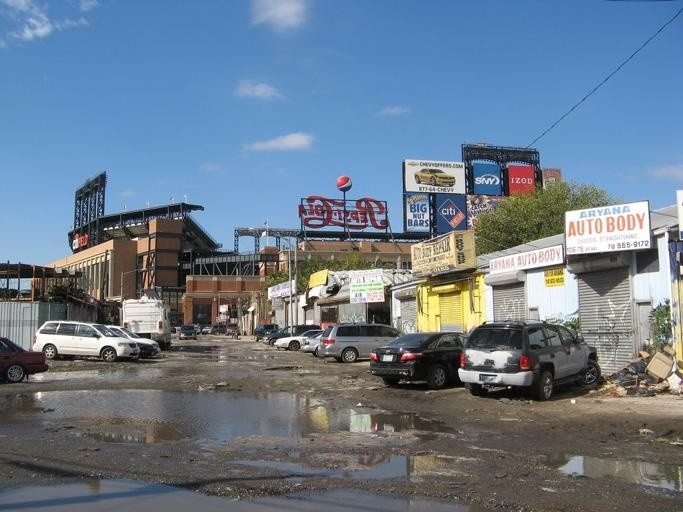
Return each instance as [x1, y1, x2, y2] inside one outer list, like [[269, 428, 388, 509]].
[[262, 325, 321, 346], [32, 320, 141, 362], [317, 322, 403, 363]]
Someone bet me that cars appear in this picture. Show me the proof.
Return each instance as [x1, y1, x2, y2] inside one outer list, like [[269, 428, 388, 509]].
[[1, 336, 50, 384], [178, 324, 198, 340], [414, 168, 456, 187], [457, 319, 602, 402], [253, 324, 279, 342], [202, 326, 212, 334], [273, 329, 323, 351], [300, 332, 324, 357], [224, 323, 240, 336], [370, 332, 469, 390], [212, 324, 226, 335], [107, 325, 162, 359], [194, 324, 201, 334], [171, 327, 176, 333]]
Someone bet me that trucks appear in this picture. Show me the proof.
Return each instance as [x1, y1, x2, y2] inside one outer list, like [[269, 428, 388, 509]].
[[118, 294, 177, 349]]
